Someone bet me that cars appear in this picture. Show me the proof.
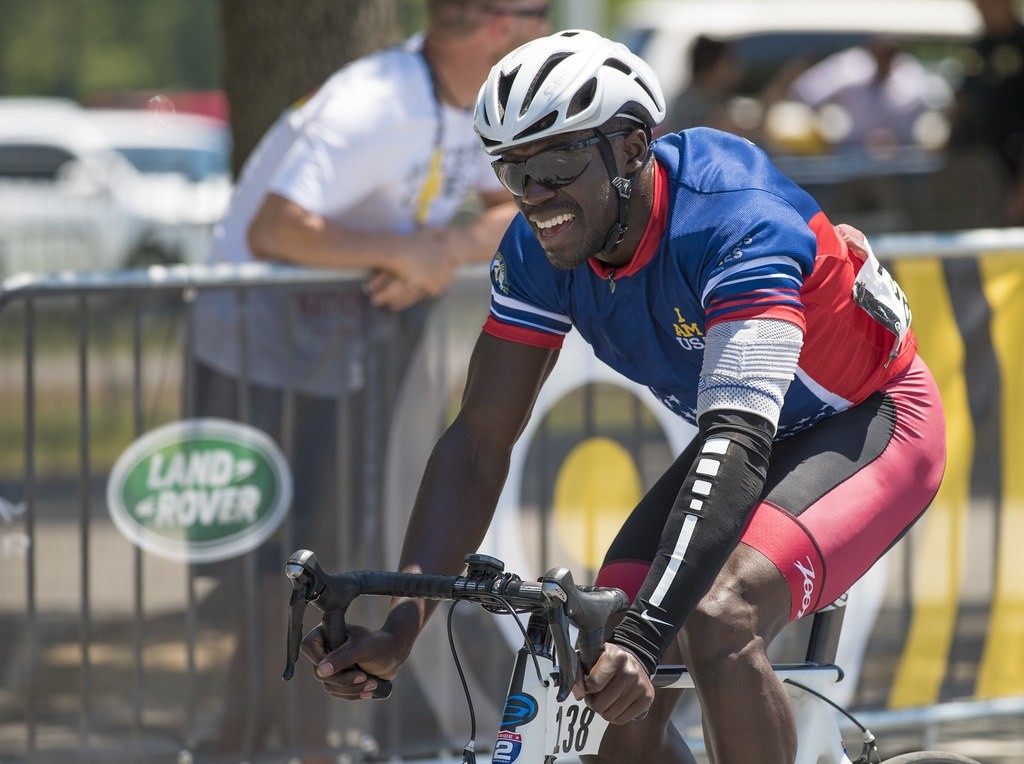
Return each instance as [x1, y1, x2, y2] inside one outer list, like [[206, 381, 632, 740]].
[[0, 89, 236, 287]]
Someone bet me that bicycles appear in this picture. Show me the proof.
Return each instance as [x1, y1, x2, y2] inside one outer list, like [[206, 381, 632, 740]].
[[282, 547, 986, 764]]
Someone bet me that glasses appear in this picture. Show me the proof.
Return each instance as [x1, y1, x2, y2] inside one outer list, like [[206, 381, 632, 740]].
[[490, 129, 631, 197]]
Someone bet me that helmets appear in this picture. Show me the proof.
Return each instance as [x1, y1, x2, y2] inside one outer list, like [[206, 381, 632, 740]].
[[470, 28, 668, 156]]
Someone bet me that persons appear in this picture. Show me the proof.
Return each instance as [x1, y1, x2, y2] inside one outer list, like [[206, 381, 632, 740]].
[[947, 0, 1023, 234], [813, 34, 950, 232], [184, 2, 552, 761], [658, 33, 758, 142], [301, 29, 949, 764]]
